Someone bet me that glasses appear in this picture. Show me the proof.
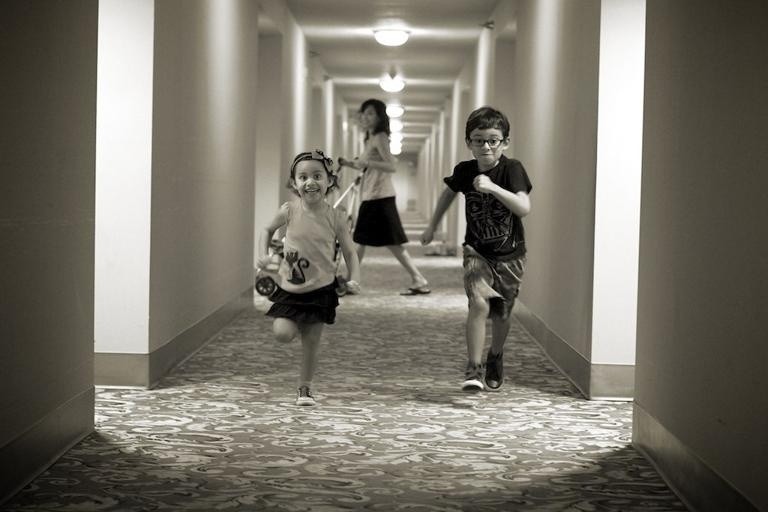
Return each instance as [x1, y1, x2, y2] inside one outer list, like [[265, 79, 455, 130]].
[[467, 136, 505, 147]]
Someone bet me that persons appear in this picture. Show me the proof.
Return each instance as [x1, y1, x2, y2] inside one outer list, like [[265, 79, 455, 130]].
[[257, 148, 358, 406], [418, 106, 532, 393], [337, 98, 430, 295]]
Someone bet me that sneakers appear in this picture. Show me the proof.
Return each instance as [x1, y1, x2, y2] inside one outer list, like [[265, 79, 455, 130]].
[[462, 363, 485, 390], [485, 349, 504, 390], [295, 385, 315, 406]]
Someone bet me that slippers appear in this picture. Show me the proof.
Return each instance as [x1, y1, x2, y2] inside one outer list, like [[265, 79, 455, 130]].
[[399, 288, 432, 296]]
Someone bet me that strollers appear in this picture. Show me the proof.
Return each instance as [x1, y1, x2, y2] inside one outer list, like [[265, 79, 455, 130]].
[[255, 157, 368, 297]]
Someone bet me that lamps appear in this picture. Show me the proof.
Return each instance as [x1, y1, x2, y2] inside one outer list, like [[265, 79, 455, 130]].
[[373, 19, 410, 156]]
